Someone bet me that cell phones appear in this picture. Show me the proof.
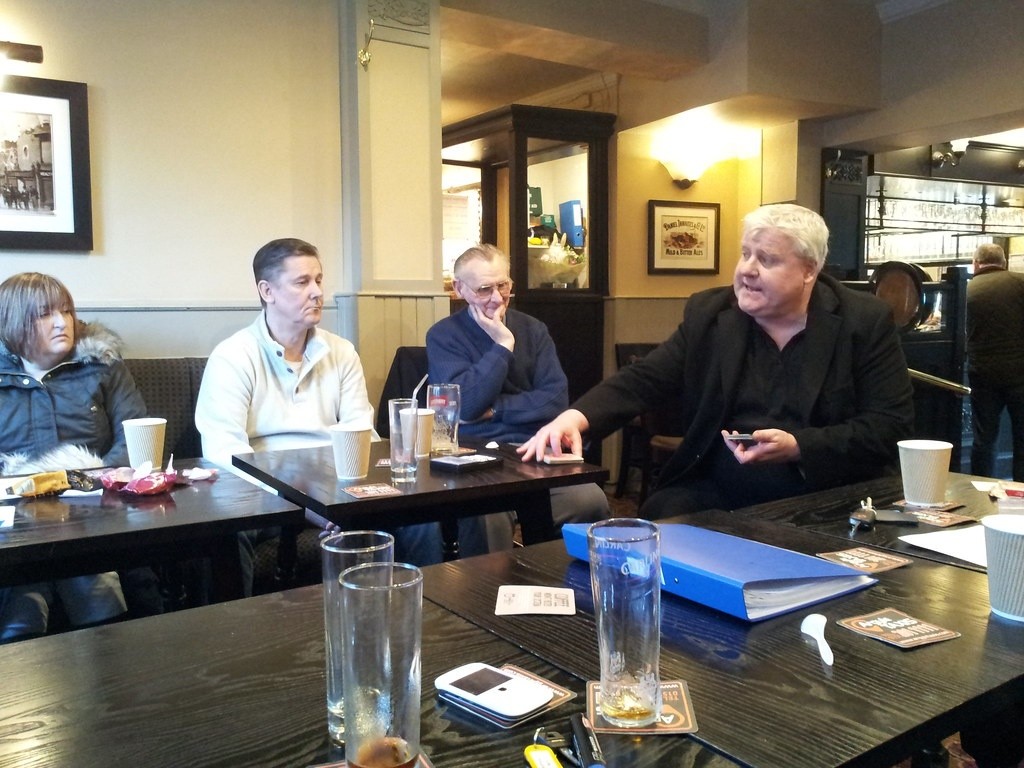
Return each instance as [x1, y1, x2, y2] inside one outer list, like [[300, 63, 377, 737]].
[[435, 662, 553, 721]]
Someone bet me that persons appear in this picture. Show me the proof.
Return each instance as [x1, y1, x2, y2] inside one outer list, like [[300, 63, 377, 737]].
[[966, 243, 1024, 484], [0, 182, 39, 210], [194, 239, 442, 599], [0, 271, 151, 646], [514, 204, 914, 525], [425, 243, 611, 558]]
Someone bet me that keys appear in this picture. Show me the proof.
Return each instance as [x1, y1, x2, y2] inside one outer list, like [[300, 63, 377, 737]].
[[848, 496, 877, 536], [537, 731, 581, 766]]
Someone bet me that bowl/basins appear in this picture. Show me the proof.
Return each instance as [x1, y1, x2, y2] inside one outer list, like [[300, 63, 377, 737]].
[[528, 245, 550, 257]]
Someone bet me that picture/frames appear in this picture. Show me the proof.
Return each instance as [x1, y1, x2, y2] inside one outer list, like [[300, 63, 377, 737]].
[[648, 199, 720, 276], [0, 74, 94, 253]]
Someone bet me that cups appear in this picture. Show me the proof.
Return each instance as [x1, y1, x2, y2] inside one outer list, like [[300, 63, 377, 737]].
[[541, 234, 551, 245], [329, 423, 371, 480], [122, 418, 168, 472], [338, 562, 423, 768], [896, 441, 953, 507], [427, 384, 461, 456], [320, 531, 394, 742], [588, 518, 660, 725], [399, 409, 435, 457], [982, 514, 1024, 622], [389, 399, 418, 483]]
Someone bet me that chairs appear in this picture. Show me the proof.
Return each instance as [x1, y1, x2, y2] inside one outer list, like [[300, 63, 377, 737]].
[[377, 345, 524, 548], [613, 343, 684, 517]]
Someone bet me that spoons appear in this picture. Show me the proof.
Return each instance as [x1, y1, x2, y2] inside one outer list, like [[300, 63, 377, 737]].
[[801, 612, 836, 667]]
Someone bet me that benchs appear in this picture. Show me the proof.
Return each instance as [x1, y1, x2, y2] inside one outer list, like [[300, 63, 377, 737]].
[[126, 357, 331, 593]]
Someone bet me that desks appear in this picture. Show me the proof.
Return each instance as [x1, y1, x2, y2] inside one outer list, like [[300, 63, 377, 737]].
[[0, 573, 743, 768], [0, 458, 303, 602], [730, 471, 1024, 575], [232, 436, 611, 575], [375, 510, 1024, 768]]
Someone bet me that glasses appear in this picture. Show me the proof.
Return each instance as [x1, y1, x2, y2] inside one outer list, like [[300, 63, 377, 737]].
[[460, 275, 514, 298]]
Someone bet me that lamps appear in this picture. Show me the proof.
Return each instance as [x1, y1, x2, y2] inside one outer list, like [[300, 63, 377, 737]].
[[931, 137, 969, 168], [658, 158, 707, 188], [0, 40, 45, 64]]
